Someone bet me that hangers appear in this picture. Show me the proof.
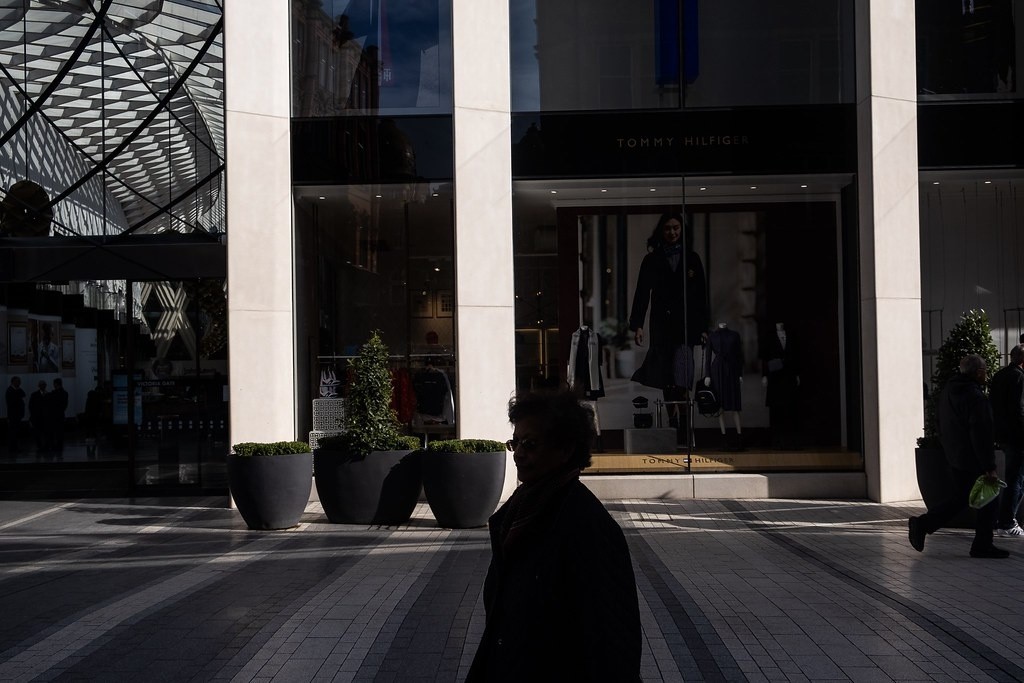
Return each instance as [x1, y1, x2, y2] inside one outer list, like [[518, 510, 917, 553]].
[[317, 353, 456, 381]]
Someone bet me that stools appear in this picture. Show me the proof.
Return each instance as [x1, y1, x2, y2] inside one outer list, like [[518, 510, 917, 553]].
[[624, 426, 678, 454]]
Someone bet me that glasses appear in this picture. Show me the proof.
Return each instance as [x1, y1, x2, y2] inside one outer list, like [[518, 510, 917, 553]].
[[505, 439, 557, 452]]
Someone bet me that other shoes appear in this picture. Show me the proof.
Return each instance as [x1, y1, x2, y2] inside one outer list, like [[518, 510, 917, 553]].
[[909, 517, 926, 552], [969, 545, 1010, 558]]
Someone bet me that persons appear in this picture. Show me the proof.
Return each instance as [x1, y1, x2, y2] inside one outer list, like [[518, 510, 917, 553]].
[[990, 343, 1024, 537], [462, 382, 641, 682], [625, 215, 712, 451], [909, 354, 1010, 560], [39, 322, 59, 373], [6, 374, 110, 459]]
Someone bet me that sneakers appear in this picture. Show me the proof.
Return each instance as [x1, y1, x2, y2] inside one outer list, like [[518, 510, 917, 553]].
[[997, 523, 1024, 538]]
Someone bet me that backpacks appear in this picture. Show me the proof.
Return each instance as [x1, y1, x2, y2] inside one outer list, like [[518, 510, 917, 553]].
[[695, 379, 724, 419]]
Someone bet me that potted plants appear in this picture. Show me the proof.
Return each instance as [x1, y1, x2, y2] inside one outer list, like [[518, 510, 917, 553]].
[[226, 441, 311, 530], [314, 327, 427, 525], [420, 439, 507, 528], [914, 308, 1005, 529]]
